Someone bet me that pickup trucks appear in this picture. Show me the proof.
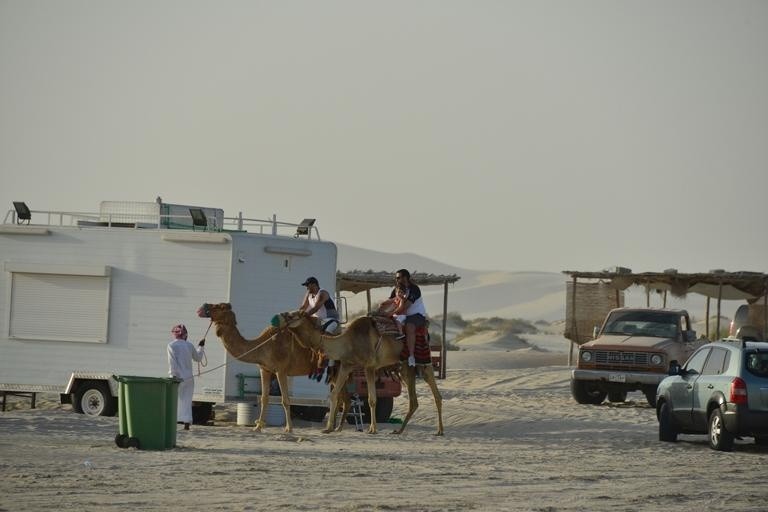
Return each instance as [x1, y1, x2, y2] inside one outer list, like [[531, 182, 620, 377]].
[[570, 306, 711, 407]]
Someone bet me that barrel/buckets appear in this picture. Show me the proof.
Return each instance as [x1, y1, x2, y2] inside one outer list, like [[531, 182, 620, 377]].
[[236, 403, 261, 428], [265, 403, 289, 427]]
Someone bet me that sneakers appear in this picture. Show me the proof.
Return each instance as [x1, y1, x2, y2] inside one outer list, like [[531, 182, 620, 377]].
[[328, 360, 335, 366], [408, 357, 415, 368], [184, 423, 189, 430], [395, 334, 405, 340]]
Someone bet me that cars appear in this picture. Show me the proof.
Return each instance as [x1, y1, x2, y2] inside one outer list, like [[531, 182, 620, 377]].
[[655, 337, 768, 450]]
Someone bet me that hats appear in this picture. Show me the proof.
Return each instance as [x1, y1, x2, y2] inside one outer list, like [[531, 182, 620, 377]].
[[171, 324, 187, 337], [301, 277, 318, 286]]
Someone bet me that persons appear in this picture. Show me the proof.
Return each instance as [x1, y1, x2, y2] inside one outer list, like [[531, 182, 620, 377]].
[[167, 324, 206, 430], [300, 276, 340, 336], [377, 269, 429, 367]]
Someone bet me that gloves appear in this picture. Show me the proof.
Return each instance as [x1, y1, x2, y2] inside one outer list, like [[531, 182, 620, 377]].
[[199, 340, 205, 346]]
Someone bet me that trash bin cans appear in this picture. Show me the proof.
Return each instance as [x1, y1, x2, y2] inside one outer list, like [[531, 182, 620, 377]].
[[113, 374, 184, 450]]
